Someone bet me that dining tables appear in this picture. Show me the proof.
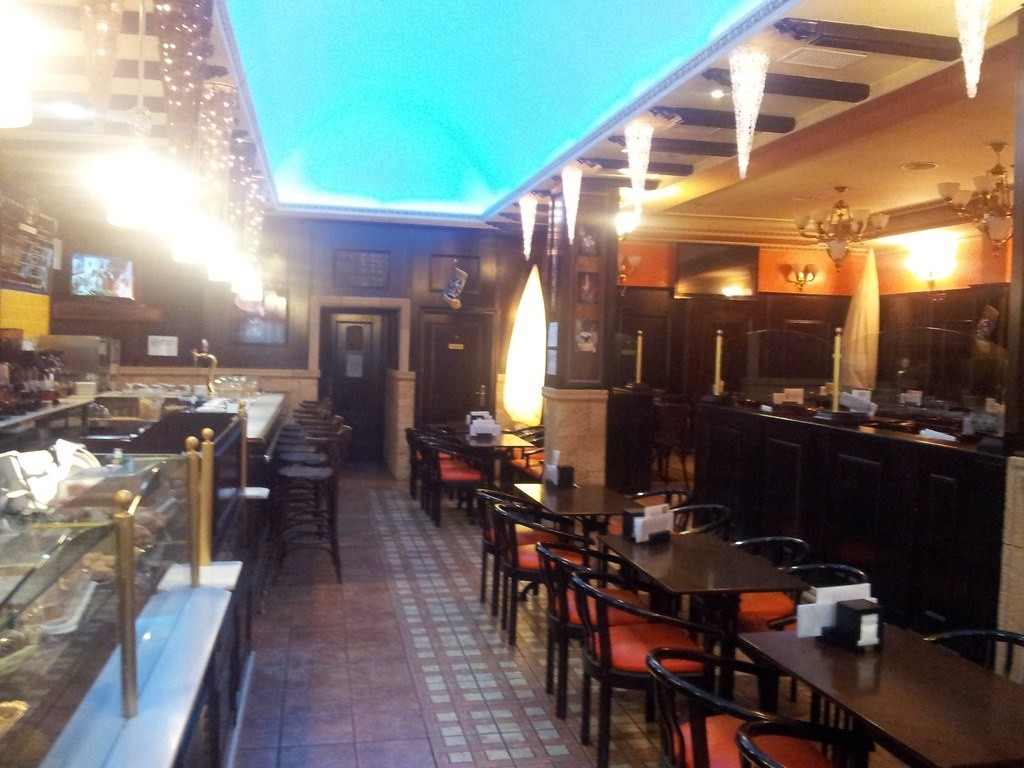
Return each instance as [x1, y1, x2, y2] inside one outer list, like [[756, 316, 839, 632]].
[[458, 430, 534, 488], [597, 532, 812, 659], [196, 390, 291, 547], [736, 622, 1024, 768], [513, 481, 651, 571]]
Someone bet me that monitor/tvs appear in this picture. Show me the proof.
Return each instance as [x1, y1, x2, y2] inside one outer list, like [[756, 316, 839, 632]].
[[674, 242, 760, 301], [70, 252, 134, 301]]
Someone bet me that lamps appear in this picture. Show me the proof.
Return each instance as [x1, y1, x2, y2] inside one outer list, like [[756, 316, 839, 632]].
[[560, 166, 582, 246], [792, 262, 822, 289], [624, 124, 655, 219], [729, 46, 773, 181], [518, 194, 541, 261], [616, 252, 640, 284], [955, 0, 990, 101], [791, 186, 890, 275], [938, 141, 1017, 258]]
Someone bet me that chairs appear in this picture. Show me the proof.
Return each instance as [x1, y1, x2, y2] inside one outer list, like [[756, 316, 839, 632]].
[[270, 397, 355, 588], [533, 540, 877, 768], [405, 427, 499, 528], [731, 533, 1024, 688], [473, 489, 595, 645], [501, 425, 545, 493], [621, 486, 734, 542], [653, 396, 696, 487]]
